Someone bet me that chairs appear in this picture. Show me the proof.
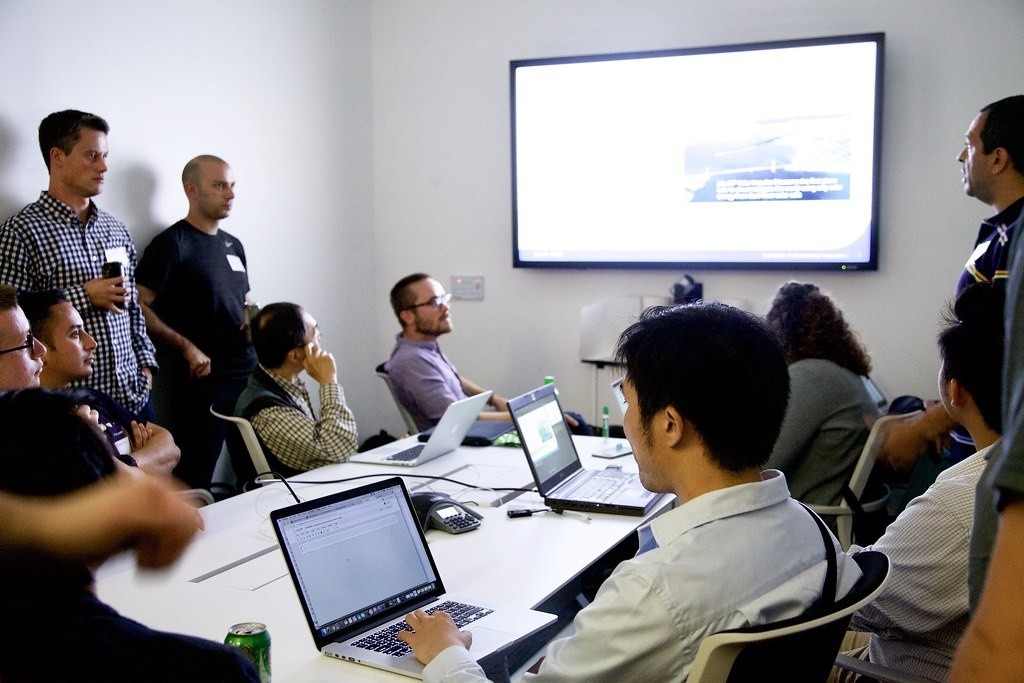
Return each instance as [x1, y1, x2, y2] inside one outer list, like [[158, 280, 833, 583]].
[[804, 409, 923, 553], [375, 362, 419, 436], [685, 550, 892, 683], [209, 401, 274, 493]]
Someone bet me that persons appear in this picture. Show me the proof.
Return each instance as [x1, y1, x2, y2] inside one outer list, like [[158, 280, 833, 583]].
[[760, 282, 883, 526], [0, 287, 263, 683], [0, 109, 159, 424], [234, 303, 408, 478], [399, 302, 864, 683], [133, 154, 257, 496], [385, 273, 594, 436], [826, 95, 1024, 683]]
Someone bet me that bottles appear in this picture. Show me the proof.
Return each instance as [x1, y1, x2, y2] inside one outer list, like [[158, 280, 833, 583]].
[[544, 376, 560, 400], [601, 407, 608, 438]]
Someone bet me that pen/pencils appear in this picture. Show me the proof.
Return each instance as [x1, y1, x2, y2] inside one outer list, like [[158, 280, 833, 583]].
[[552, 507, 591, 520]]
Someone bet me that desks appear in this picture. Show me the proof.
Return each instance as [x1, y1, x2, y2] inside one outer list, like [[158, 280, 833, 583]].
[[92, 435, 677, 683], [580, 359, 627, 435]]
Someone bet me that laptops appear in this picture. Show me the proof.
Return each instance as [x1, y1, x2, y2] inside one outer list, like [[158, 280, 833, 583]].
[[349, 390, 494, 467], [507, 383, 666, 515], [269, 477, 560, 680], [416, 419, 515, 447]]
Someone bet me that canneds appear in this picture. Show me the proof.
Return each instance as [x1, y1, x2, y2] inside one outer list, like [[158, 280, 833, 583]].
[[223, 622, 271, 683], [102, 261, 128, 309]]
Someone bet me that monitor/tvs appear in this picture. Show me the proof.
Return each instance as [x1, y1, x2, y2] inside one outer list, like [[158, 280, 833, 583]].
[[510, 32, 887, 271]]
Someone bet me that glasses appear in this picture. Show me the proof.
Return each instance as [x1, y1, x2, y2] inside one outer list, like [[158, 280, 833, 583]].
[[403, 292, 451, 312], [0, 326, 34, 358]]
